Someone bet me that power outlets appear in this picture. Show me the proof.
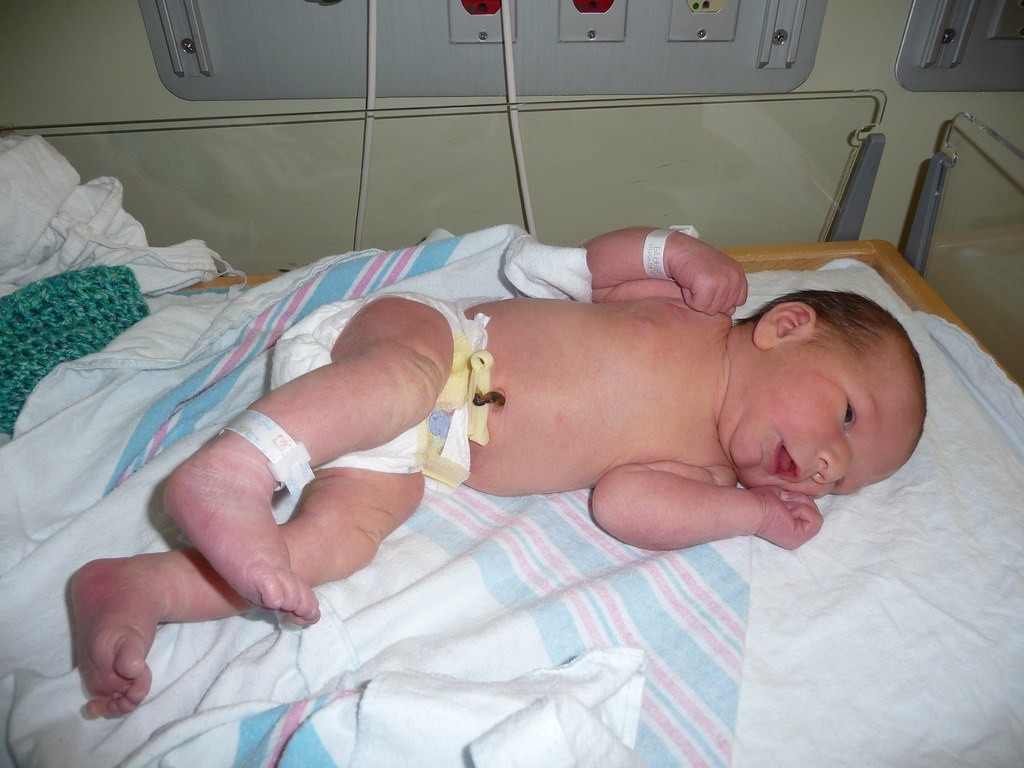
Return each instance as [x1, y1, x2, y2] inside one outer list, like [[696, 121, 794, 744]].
[[988, 0, 1024, 41], [446, 0, 519, 45], [666, 0, 740, 42], [558, 0, 628, 43]]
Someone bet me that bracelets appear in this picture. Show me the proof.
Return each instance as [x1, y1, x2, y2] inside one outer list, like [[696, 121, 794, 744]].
[[643, 229, 679, 282]]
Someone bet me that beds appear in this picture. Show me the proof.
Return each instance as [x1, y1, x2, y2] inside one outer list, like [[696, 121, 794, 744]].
[[0, 237, 1024, 768]]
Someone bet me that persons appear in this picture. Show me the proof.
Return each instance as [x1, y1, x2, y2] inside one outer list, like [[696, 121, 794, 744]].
[[70, 226, 926, 719]]
